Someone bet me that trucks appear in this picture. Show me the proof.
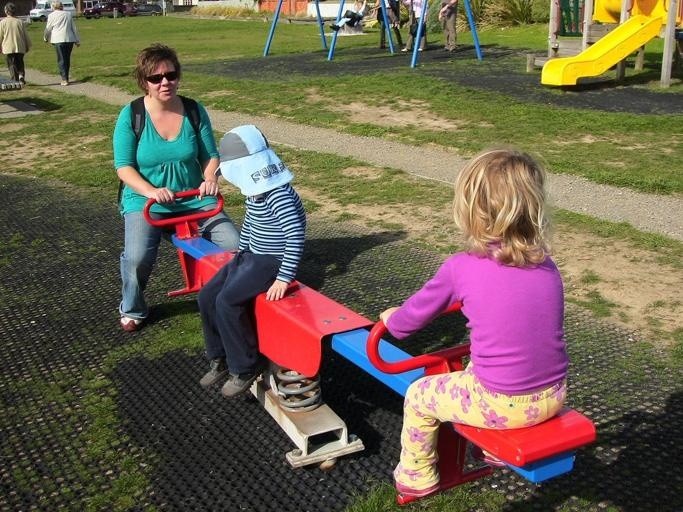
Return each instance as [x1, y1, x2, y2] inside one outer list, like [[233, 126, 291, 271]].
[[29, 0, 76, 22]]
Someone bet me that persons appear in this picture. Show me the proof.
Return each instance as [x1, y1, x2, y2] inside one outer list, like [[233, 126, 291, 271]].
[[379, 147, 570, 497], [0, 3, 31, 83], [331, 1, 461, 54], [191, 126, 306, 397], [111, 43, 241, 336], [41, 1, 80, 86]]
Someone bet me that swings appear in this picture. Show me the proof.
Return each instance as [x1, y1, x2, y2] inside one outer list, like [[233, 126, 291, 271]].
[[344, 0, 368, 28], [390, 1, 409, 25]]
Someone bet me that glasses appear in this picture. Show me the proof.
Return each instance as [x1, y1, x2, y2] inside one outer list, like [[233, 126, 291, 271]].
[[144, 70, 178, 85]]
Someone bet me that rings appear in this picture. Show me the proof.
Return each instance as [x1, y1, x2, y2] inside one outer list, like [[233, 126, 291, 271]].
[[209, 192, 214, 196]]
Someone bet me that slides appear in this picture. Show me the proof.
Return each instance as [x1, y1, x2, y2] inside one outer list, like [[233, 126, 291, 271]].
[[541, 14, 663, 86]]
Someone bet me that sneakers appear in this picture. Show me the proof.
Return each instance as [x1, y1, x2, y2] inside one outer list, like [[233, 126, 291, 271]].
[[120, 315, 144, 332], [221, 368, 262, 398], [18, 75, 26, 85], [200, 358, 228, 390], [60, 81, 68, 86], [471, 446, 507, 468], [395, 482, 440, 498]]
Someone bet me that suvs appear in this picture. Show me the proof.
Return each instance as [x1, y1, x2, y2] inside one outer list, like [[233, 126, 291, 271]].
[[81, 0, 167, 19]]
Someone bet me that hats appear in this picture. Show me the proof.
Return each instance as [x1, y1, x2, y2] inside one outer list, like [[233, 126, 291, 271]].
[[218, 124, 295, 197]]
